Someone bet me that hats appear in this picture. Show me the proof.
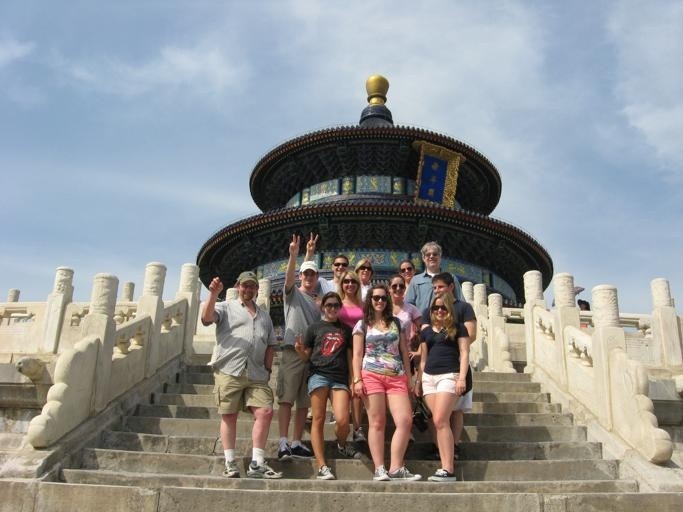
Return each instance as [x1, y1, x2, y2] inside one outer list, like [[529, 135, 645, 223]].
[[237, 271, 258, 286], [299, 261, 319, 274]]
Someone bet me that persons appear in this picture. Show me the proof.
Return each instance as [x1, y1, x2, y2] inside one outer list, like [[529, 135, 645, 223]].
[[578, 298, 592, 329], [200, 271, 284, 478], [278, 232, 478, 460], [352, 284, 422, 480], [414, 292, 470, 481], [295, 291, 361, 481]]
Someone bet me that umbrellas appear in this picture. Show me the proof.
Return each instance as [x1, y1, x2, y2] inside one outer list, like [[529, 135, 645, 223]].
[[552, 286, 585, 306]]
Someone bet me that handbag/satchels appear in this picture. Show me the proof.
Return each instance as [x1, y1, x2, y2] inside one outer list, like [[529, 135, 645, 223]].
[[412, 396, 429, 434]]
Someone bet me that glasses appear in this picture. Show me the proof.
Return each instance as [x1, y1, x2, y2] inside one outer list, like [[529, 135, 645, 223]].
[[333, 263, 348, 267], [432, 305, 447, 310], [392, 284, 404, 290], [401, 267, 412, 273], [371, 295, 388, 302], [360, 266, 372, 271], [344, 279, 357, 284], [324, 303, 340, 308]]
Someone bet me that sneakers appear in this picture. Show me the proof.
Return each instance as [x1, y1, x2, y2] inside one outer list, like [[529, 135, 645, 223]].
[[222, 409, 465, 482]]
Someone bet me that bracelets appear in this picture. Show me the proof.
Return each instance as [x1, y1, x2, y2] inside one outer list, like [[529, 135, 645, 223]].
[[266, 367, 273, 373]]
